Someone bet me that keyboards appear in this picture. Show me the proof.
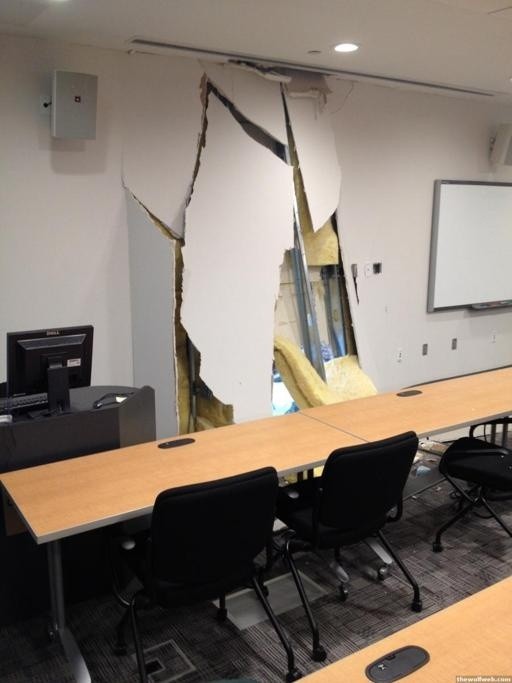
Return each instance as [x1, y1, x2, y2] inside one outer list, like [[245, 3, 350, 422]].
[[0, 393, 47, 415]]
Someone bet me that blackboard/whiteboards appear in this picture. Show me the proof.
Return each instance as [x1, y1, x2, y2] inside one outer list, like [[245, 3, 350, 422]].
[[428, 180, 512, 313]]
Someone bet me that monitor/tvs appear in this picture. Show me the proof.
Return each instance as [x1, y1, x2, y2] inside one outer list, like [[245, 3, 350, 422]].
[[7, 325, 93, 419]]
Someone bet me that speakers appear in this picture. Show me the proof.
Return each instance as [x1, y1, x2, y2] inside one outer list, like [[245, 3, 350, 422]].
[[50, 69, 97, 140]]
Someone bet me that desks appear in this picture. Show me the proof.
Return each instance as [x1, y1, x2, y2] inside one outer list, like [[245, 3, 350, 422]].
[[0, 363, 512, 683]]
